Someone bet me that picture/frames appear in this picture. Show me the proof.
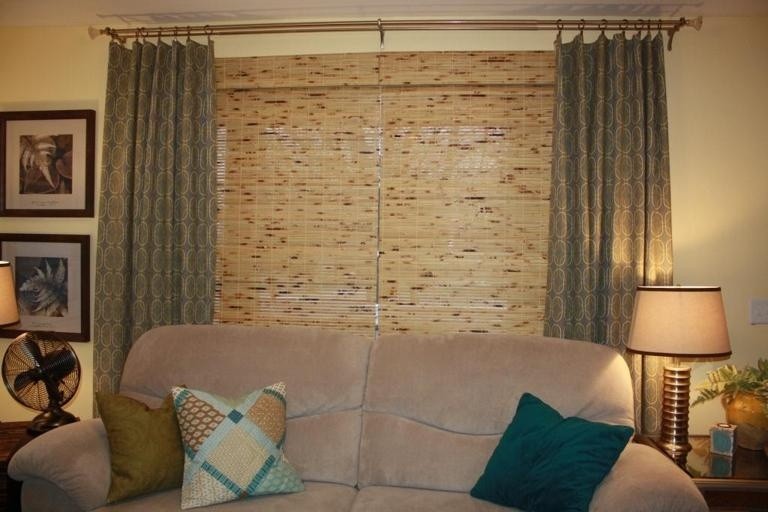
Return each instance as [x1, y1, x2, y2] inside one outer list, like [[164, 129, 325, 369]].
[[0, 233, 91, 342], [0, 108, 96, 217]]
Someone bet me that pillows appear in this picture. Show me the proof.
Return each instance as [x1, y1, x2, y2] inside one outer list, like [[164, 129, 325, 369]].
[[95, 383, 188, 504], [167, 382, 305, 511], [469, 393, 635, 512]]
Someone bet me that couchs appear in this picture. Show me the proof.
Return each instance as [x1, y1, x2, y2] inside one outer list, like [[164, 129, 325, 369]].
[[6, 324, 709, 512]]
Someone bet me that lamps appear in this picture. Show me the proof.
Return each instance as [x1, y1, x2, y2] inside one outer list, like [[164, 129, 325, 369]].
[[627, 285, 732, 464], [0, 261, 19, 327]]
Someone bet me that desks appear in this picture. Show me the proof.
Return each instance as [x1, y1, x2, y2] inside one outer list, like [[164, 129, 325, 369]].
[[0, 417, 79, 512], [634, 434, 767, 512]]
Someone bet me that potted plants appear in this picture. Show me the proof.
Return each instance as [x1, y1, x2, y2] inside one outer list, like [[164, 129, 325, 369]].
[[690, 358, 768, 450]]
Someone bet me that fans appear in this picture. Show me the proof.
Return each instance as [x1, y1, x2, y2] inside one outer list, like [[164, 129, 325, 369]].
[[1, 331, 81, 434]]
